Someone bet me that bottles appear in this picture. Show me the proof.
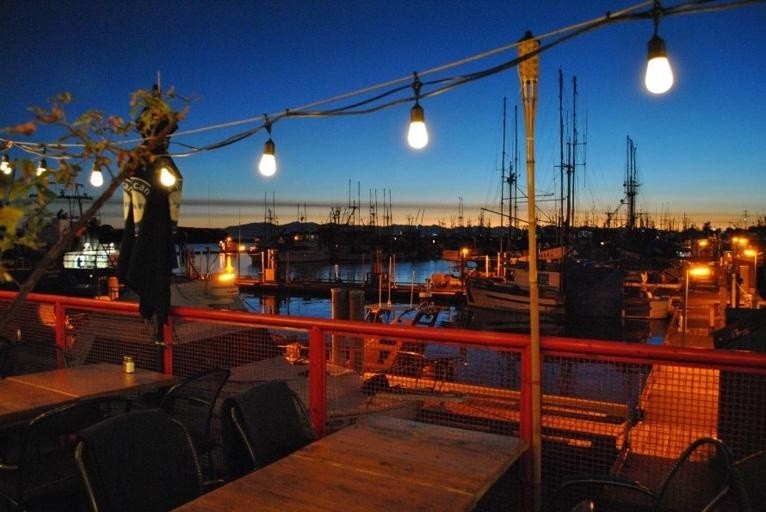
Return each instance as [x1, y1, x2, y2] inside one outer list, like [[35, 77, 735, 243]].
[[122, 356, 134, 373]]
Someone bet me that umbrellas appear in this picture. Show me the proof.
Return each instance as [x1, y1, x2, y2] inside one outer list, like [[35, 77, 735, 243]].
[[113, 88, 182, 377]]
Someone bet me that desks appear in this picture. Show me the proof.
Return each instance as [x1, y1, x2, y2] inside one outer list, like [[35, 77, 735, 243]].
[[7, 360, 178, 407], [290, 413, 533, 505], [169, 455, 478, 511], [0, 377, 81, 435]]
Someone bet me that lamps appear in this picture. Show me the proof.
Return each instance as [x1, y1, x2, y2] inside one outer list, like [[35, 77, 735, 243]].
[[0, 149, 13, 175], [257, 116, 283, 178], [643, 5, 673, 98], [88, 153, 105, 190], [150, 146, 176, 188], [407, 73, 429, 152], [36, 150, 49, 178]]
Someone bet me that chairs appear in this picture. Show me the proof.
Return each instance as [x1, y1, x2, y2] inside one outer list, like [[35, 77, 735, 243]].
[[73, 407, 226, 512], [220, 376, 320, 472], [2, 395, 132, 512], [130, 366, 235, 470], [539, 433, 757, 511]]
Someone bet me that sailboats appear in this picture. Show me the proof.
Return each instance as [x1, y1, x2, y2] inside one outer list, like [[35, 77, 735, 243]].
[[30, 179, 121, 273]]
